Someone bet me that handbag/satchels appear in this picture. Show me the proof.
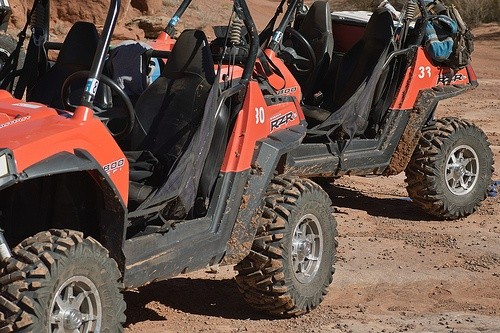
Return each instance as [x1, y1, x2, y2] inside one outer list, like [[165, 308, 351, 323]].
[[422, 15, 457, 62], [450, 30, 475, 66]]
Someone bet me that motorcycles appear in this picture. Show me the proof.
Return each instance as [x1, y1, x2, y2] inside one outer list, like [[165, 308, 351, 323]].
[[0, 0, 339, 333], [252, 0, 495, 218]]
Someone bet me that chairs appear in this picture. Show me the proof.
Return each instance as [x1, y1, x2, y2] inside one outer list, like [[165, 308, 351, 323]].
[[28, 21, 113, 127], [301, 7, 397, 127], [283, 1, 334, 97], [124, 28, 229, 214]]
